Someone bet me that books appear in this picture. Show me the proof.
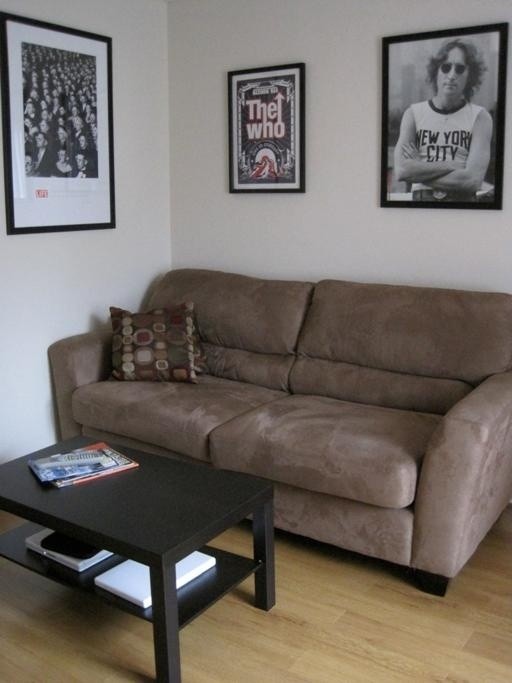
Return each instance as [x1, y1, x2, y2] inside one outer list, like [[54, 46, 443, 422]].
[[57, 440, 140, 489], [28, 447, 120, 485]]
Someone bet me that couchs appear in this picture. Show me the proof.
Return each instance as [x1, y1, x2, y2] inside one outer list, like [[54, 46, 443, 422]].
[[47, 269, 512, 598]]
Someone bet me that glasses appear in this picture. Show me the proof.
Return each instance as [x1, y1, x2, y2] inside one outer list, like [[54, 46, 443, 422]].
[[441, 62, 465, 75]]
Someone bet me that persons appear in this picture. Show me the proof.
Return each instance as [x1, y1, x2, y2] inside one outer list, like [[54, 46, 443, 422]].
[[20, 38, 99, 178], [394, 38, 495, 203]]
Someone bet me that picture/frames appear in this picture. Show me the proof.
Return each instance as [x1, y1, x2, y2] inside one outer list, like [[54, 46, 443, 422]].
[[379, 20, 509, 213], [1, 7, 117, 237], [229, 60, 307, 192]]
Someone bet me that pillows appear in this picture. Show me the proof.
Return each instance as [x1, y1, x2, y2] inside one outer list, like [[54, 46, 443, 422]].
[[107, 301, 212, 386]]
